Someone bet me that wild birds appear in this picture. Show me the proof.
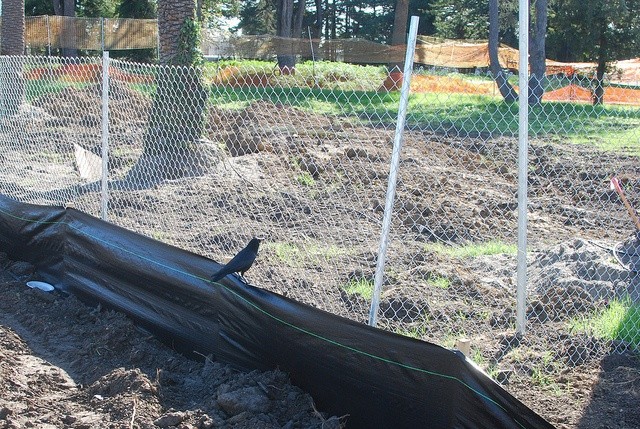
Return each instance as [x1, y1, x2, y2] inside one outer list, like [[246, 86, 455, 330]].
[[209, 237, 266, 286]]
